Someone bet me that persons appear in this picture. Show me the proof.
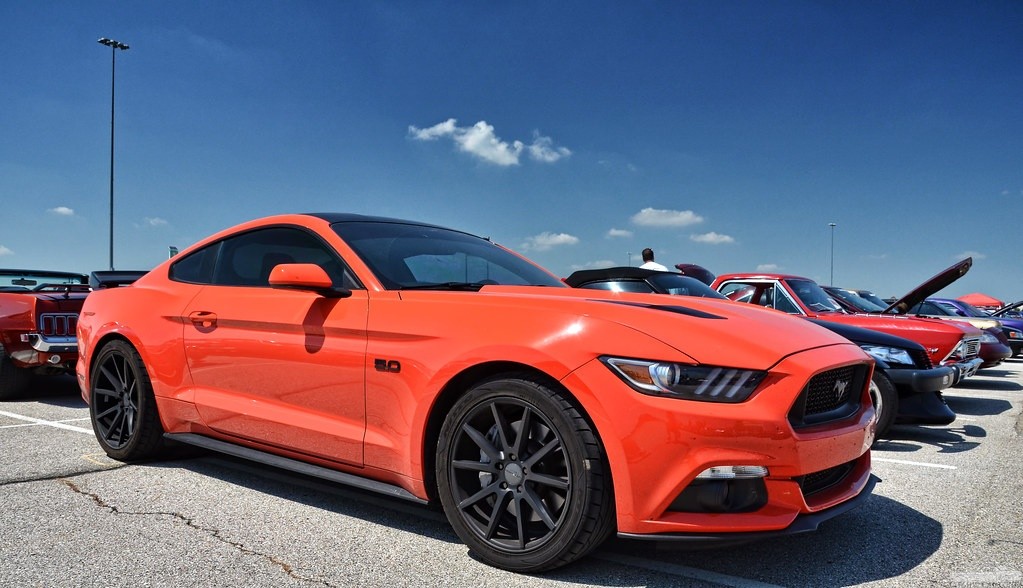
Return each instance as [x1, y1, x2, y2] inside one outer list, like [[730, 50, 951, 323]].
[[638, 248, 669, 272]]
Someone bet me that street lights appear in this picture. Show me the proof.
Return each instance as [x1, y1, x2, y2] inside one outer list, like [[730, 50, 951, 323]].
[[97, 36, 128, 264], [826, 221, 836, 287]]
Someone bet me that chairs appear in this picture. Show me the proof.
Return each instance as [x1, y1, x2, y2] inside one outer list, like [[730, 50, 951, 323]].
[[776, 292, 805, 314]]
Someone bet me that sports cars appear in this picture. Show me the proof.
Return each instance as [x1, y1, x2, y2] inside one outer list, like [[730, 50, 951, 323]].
[[550, 255, 1023, 427], [74, 211, 883, 578]]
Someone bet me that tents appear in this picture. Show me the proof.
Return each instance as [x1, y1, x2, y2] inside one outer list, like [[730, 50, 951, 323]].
[[957, 292, 1005, 319]]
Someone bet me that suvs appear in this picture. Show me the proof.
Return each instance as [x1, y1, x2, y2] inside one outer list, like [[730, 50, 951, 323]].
[[0, 269, 95, 402]]
